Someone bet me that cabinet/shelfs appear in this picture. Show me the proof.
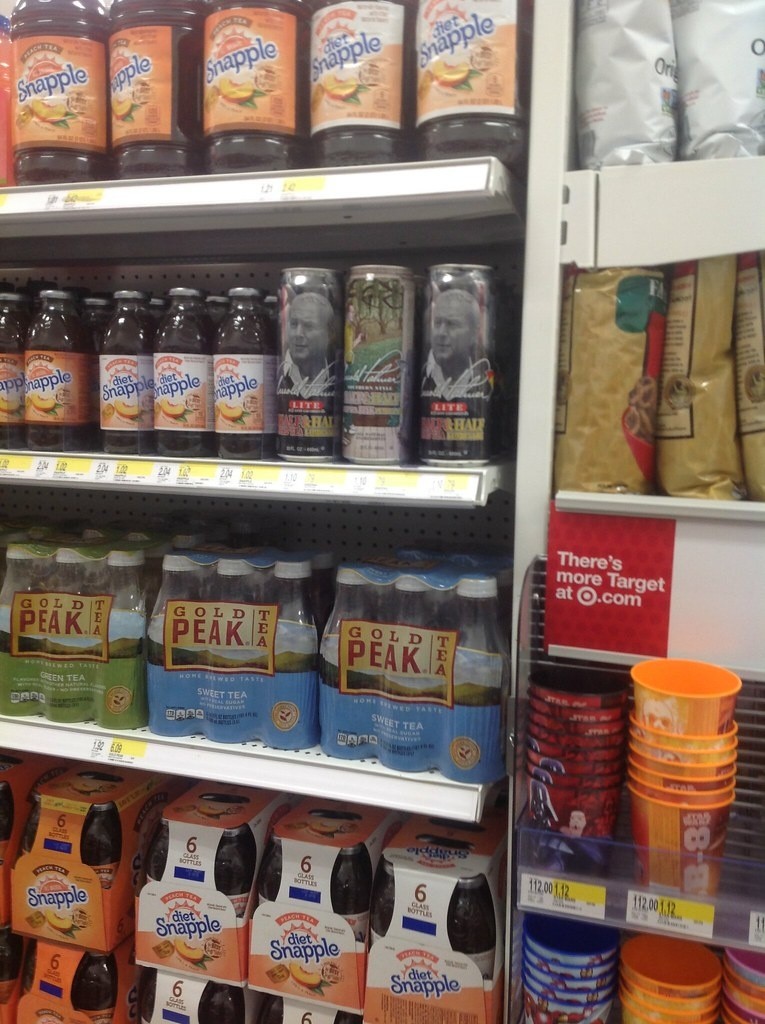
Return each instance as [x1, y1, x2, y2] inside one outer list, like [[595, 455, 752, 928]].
[[511, 0, 765, 1024], [0, 156, 520, 824]]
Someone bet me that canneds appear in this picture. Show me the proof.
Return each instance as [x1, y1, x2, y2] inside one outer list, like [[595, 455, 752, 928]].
[[279, 265, 521, 461]]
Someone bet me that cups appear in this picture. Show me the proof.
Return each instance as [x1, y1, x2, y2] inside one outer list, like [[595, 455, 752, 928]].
[[526, 659, 743, 912], [520, 915, 765, 1024]]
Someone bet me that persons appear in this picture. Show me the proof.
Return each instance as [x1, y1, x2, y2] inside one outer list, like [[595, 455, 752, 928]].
[[425, 289, 480, 401], [283, 292, 335, 398]]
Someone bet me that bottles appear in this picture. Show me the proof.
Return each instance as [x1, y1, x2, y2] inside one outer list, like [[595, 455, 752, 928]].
[[0, 287, 278, 467], [2, 0, 530, 190], [0, 511, 514, 1024]]
